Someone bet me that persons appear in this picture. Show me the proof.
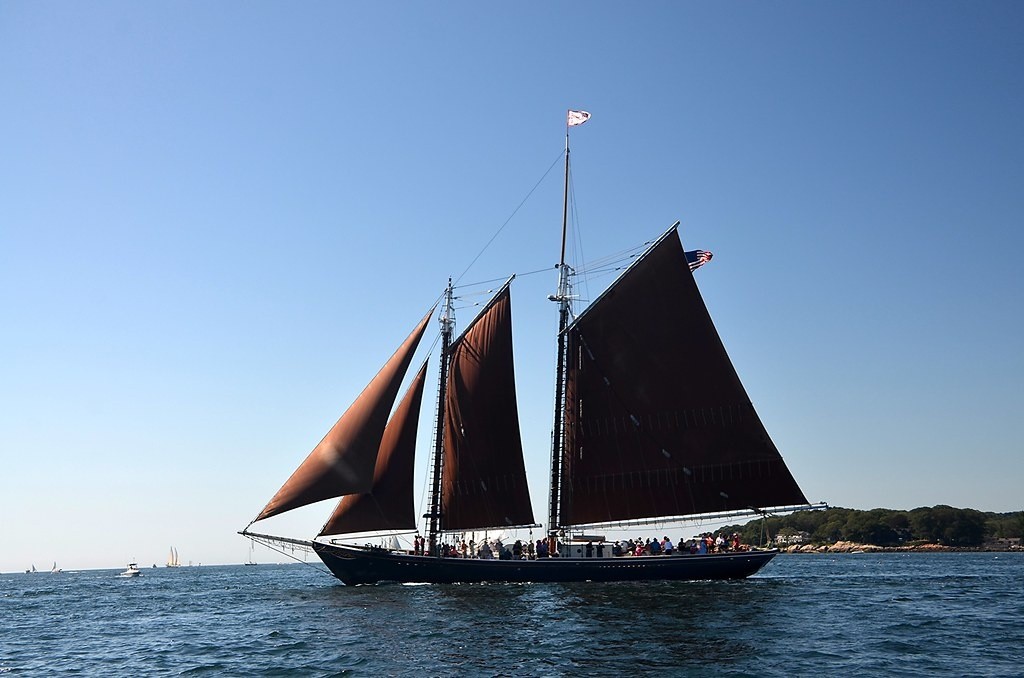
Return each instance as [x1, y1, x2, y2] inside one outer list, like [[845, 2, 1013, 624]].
[[411, 533, 754, 562]]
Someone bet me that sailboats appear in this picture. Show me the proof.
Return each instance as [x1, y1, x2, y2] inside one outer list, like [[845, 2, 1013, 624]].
[[198, 562, 202, 566], [50, 562, 62, 575], [166, 545, 181, 568], [25, 565, 39, 575], [244, 545, 257, 566], [151, 564, 159, 570], [238, 108, 831, 587], [188, 561, 194, 568]]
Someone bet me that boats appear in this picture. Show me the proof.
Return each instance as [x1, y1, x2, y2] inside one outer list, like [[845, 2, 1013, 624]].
[[121, 561, 143, 578]]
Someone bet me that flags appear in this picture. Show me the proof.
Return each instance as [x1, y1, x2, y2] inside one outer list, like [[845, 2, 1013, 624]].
[[568, 108, 592, 128], [683, 249, 713, 275]]
